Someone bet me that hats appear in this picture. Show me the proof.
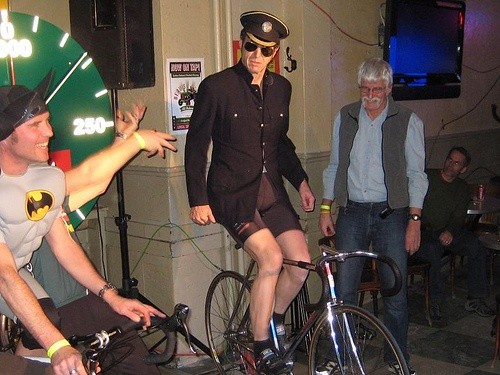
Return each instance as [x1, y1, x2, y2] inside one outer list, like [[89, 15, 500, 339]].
[[239, 10, 291, 42], [0, 67, 54, 143]]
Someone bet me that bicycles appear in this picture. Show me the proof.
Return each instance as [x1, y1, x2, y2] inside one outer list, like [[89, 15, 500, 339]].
[[0, 302, 197, 375], [205, 209, 410, 375]]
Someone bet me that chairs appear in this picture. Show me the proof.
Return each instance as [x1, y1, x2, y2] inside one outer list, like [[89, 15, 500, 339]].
[[317, 232, 456, 339], [478, 228, 500, 287]]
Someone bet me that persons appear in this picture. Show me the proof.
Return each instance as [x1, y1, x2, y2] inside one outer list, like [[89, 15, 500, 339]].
[[0, 75, 178, 375], [317, 57, 495, 375], [185, 11, 315, 375]]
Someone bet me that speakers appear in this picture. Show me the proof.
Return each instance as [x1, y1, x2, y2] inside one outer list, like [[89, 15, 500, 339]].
[[68, 0, 156, 91]]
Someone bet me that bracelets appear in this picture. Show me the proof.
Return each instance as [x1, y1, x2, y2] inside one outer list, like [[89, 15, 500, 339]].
[[47, 338, 70, 359], [133, 131, 146, 151], [117, 132, 128, 140], [320, 211, 331, 214], [320, 205, 330, 210]]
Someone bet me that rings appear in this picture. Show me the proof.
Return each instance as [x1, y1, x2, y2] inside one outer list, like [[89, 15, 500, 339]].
[[70, 369, 77, 375], [152, 129, 156, 132]]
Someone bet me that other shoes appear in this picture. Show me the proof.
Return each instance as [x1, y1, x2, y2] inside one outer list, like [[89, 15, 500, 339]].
[[425, 305, 443, 322], [465, 298, 494, 316]]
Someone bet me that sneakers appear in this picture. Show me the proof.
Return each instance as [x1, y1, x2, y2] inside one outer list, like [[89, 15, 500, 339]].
[[315, 361, 348, 375], [253, 324, 294, 375], [388, 363, 415, 375]]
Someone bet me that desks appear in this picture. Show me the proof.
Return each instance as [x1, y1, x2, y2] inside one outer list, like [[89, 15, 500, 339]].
[[466, 196, 500, 232]]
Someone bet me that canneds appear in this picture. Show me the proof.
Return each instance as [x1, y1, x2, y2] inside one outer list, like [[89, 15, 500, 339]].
[[477, 184, 486, 200]]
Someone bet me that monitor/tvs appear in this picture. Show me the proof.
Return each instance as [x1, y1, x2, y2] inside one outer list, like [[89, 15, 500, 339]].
[[384, 0, 465, 102]]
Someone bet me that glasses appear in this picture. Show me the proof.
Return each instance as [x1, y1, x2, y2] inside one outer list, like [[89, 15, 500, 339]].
[[245, 42, 274, 57]]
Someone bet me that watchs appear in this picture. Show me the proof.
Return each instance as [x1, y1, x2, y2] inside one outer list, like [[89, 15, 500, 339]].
[[409, 214, 421, 221], [99, 283, 118, 301]]
